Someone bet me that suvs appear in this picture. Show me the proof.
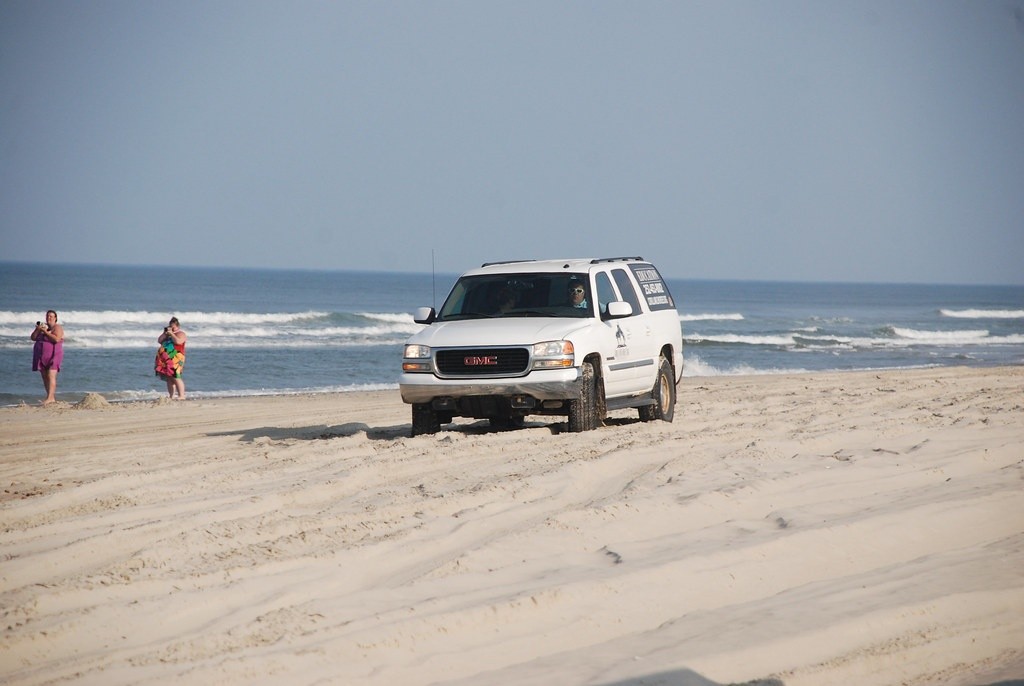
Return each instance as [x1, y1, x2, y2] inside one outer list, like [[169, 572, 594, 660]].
[[399, 256, 685, 435]]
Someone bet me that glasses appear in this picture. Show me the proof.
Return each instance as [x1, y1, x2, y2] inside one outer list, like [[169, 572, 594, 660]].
[[568, 288, 584, 295]]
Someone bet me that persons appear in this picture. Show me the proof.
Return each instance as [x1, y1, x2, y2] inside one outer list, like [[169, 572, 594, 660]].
[[496, 287, 519, 314], [32, 311, 64, 404], [568, 279, 606, 312], [155, 317, 186, 400]]
